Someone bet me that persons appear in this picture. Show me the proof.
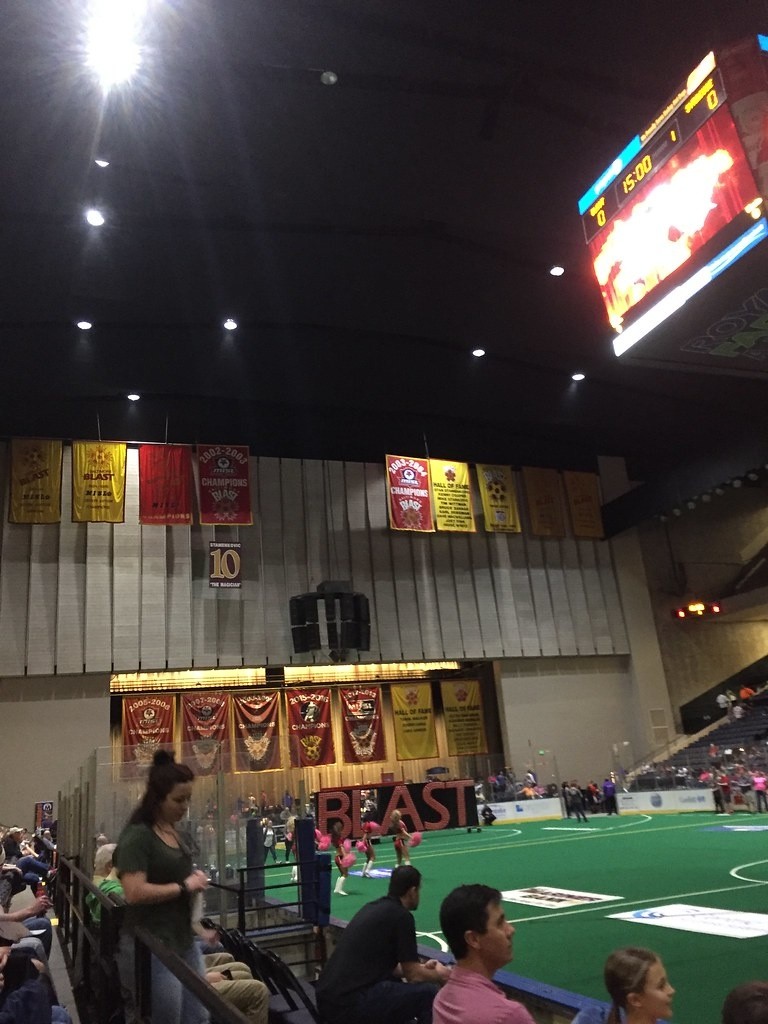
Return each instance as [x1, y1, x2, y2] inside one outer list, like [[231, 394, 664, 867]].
[[286, 810, 413, 896], [315, 866, 535, 1024], [697, 743, 768, 813], [665, 765, 697, 786], [0, 818, 269, 1024], [715, 684, 756, 720], [722, 979, 768, 1024], [641, 762, 649, 774], [357, 781, 378, 819], [562, 778, 617, 823], [114, 750, 211, 1023], [205, 788, 315, 863], [572, 948, 674, 1024], [475, 769, 546, 826]]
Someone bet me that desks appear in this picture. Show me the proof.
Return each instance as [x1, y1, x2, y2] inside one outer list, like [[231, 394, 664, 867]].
[[615, 788, 721, 813], [476, 797, 567, 826]]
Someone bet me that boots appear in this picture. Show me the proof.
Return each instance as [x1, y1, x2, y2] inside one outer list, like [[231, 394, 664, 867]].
[[334, 876, 348, 896], [361, 862, 367, 878], [291, 865, 298, 882], [365, 860, 374, 879]]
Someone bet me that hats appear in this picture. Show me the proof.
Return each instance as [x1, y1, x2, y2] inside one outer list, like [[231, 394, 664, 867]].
[[10, 827, 23, 835]]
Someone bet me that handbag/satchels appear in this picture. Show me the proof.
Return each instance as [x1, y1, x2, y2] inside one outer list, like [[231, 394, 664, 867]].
[[179, 831, 203, 926]]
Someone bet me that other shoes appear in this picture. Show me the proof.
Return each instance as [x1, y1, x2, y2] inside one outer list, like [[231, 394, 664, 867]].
[[395, 864, 400, 869], [46, 866, 61, 883], [404, 861, 410, 866], [275, 860, 282, 864], [285, 860, 291, 863]]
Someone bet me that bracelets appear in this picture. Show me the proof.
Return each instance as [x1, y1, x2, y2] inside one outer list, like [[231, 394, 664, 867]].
[[178, 879, 188, 895]]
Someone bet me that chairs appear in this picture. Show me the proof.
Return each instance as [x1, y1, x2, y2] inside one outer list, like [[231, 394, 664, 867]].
[[631, 689, 768, 788], [200, 918, 320, 1024], [0, 856, 19, 906]]
